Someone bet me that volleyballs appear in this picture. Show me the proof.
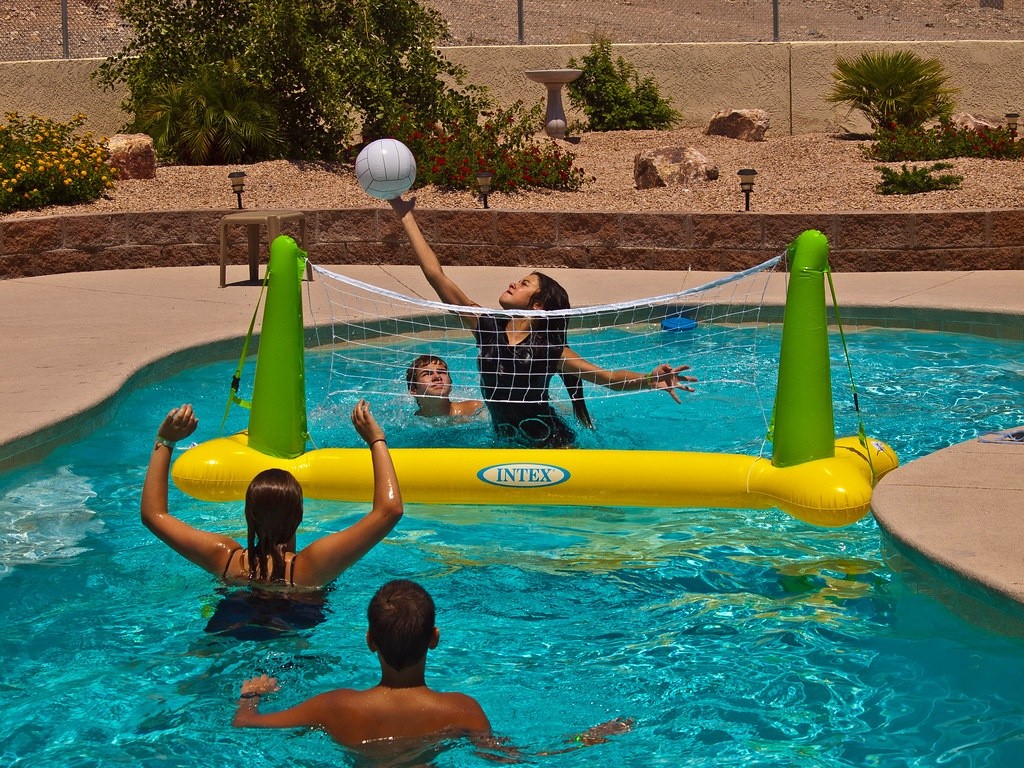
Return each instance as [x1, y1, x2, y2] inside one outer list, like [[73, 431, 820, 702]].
[[354, 137, 418, 201]]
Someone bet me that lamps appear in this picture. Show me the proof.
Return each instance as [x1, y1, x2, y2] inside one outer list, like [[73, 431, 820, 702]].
[[227, 170, 247, 195], [737, 169, 759, 194]]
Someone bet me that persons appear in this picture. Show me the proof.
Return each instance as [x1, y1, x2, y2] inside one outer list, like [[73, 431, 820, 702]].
[[389, 196, 697, 449], [406, 355, 486, 423], [142, 397, 402, 638], [231, 578, 635, 768]]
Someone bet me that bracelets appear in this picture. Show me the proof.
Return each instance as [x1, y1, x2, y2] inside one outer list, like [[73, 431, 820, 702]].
[[240, 691, 261, 698], [370, 439, 387, 450], [156, 435, 175, 448], [645, 374, 653, 391]]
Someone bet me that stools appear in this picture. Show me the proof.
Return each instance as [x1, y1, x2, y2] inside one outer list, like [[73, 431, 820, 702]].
[[219, 211, 314, 288]]
